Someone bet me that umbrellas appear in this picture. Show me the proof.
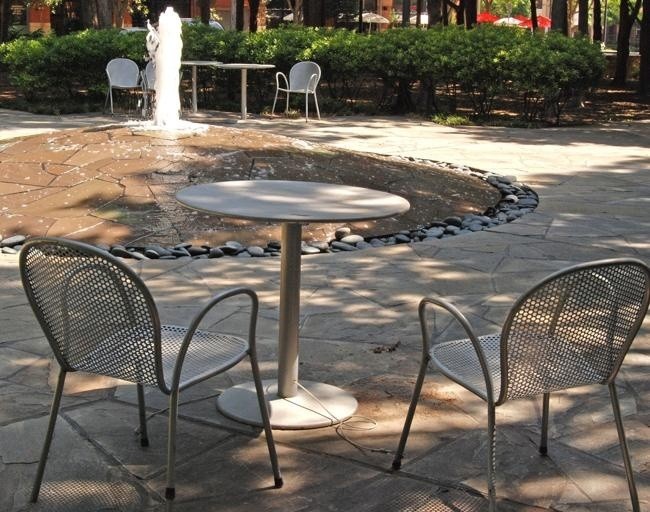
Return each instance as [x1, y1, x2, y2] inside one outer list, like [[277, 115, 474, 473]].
[[409, 13, 429, 28], [352, 12, 390, 35], [283, 13, 303, 21], [476, 11, 553, 29]]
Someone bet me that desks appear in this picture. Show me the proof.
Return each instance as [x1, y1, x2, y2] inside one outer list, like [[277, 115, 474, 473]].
[[174, 180, 411, 431], [180, 60, 276, 123]]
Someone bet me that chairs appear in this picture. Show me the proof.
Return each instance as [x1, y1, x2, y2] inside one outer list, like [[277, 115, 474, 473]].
[[390, 257, 649, 512], [272, 61, 322, 122], [104, 58, 156, 118], [18, 237, 283, 502]]
